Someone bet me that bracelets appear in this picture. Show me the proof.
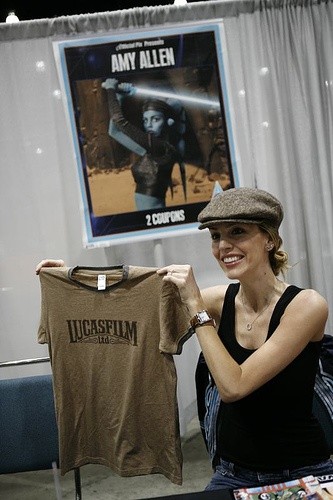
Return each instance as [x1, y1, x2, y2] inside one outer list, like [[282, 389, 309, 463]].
[[194, 318, 217, 329]]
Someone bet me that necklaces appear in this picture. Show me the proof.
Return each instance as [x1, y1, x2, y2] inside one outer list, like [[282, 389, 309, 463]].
[[241, 286, 275, 330]]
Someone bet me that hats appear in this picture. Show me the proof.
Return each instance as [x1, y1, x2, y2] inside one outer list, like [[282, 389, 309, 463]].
[[198, 187, 284, 230]]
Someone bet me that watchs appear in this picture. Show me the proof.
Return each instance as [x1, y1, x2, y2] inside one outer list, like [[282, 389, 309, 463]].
[[190, 309, 213, 329]]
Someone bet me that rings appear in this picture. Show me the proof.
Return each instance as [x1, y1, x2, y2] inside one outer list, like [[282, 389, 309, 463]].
[[168, 271, 173, 276]]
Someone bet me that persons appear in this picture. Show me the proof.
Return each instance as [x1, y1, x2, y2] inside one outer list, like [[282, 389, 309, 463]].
[[35, 187, 333, 492], [104, 78, 186, 211]]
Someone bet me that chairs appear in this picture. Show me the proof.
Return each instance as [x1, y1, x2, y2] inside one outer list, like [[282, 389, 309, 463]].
[[0, 358, 85, 500]]
